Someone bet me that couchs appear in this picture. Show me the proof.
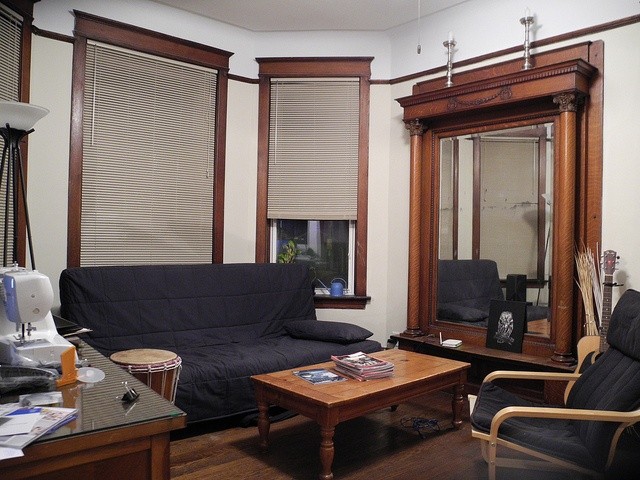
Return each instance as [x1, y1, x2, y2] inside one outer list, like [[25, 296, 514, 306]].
[[60, 262, 384, 426], [435, 259, 548, 327]]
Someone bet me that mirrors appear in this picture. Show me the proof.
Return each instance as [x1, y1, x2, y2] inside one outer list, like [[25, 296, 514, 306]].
[[427, 109, 558, 346]]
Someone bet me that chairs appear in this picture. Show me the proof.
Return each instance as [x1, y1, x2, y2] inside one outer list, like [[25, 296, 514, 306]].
[[466, 288, 639, 480]]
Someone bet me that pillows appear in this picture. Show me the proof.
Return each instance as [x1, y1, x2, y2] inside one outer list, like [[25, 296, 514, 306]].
[[438, 302, 487, 322], [281, 313, 373, 344]]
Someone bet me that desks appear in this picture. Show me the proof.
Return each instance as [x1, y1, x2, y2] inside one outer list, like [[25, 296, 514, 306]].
[[0, 334, 187, 479]]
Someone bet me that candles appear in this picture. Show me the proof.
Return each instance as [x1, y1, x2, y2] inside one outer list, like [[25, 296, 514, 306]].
[[446, 31, 454, 41], [525, 6, 530, 16]]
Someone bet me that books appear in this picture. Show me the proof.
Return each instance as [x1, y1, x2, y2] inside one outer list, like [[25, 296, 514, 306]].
[[0, 402, 79, 449], [292, 368, 347, 386], [330, 350, 394, 382]]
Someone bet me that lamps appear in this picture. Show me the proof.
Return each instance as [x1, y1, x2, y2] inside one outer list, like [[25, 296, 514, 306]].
[[0, 98, 50, 268]]
